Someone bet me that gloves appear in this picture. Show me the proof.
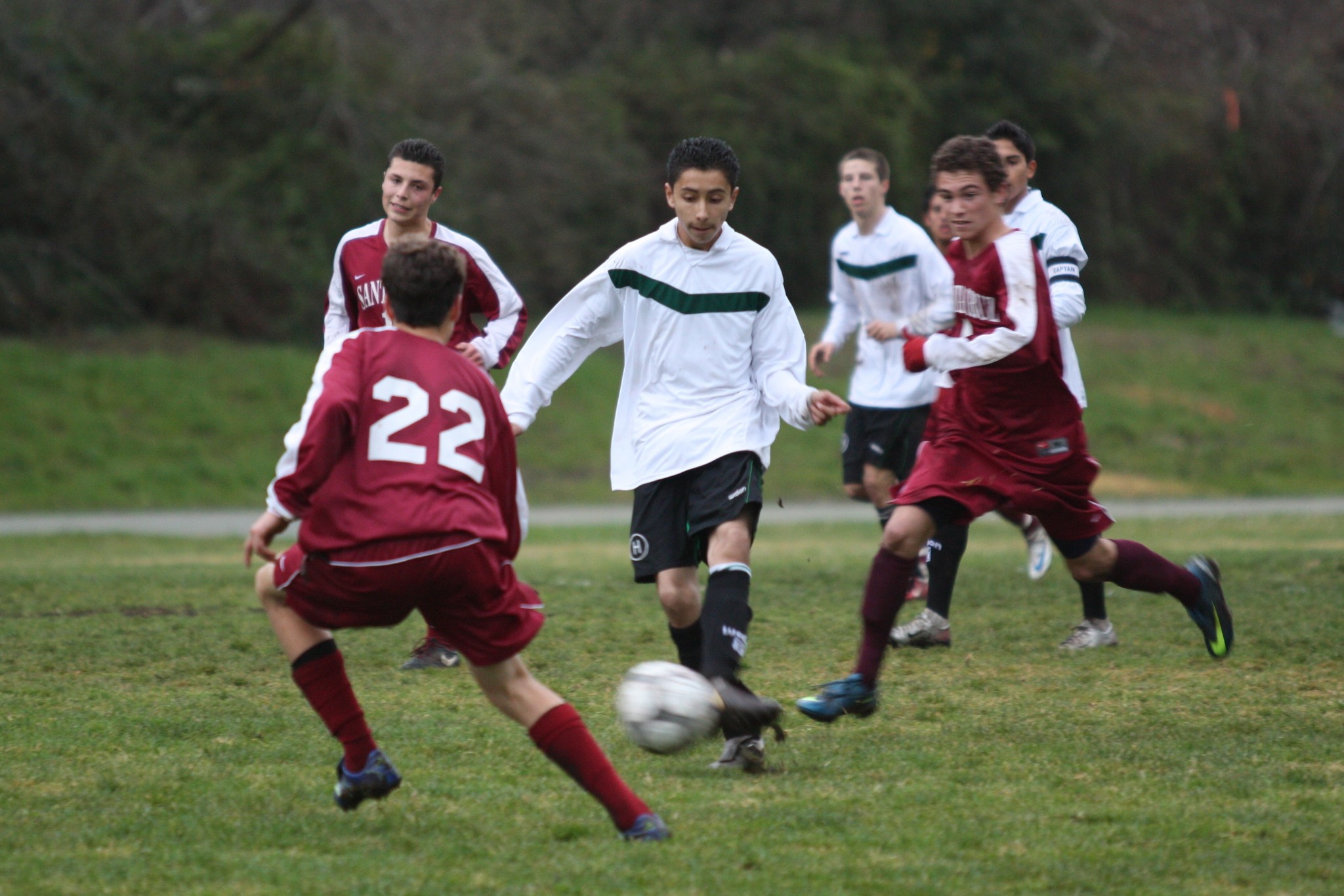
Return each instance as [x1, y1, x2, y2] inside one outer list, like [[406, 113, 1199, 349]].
[[902, 327, 926, 372]]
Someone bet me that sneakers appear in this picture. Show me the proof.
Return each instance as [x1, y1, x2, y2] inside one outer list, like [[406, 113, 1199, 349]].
[[708, 736, 765, 771], [1058, 621, 1118, 649], [1025, 525, 1051, 579], [398, 636, 462, 669], [888, 613, 951, 646], [795, 674, 876, 722], [619, 811, 672, 844], [904, 578, 930, 599], [332, 747, 400, 812], [1182, 552, 1234, 657]]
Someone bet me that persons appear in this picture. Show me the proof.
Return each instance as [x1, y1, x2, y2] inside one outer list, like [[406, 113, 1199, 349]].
[[498, 136, 851, 772], [797, 138, 1235, 724], [808, 122, 1090, 601], [245, 237, 675, 846], [322, 140, 529, 674]]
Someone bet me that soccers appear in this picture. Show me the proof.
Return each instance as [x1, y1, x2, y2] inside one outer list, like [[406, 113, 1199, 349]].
[[615, 659, 726, 755]]
